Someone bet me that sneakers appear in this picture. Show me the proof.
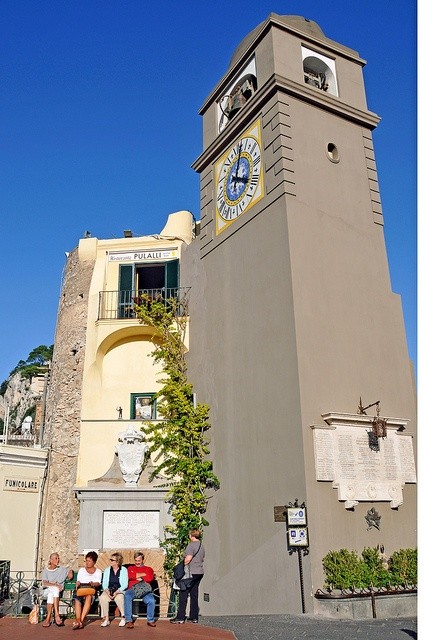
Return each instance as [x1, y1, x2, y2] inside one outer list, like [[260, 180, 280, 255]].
[[169, 618, 185, 624], [118, 619, 125, 626], [187, 617, 198, 623], [100, 620, 110, 627]]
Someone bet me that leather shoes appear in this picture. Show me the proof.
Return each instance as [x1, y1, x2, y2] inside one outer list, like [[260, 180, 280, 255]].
[[127, 622, 134, 628], [147, 622, 156, 627]]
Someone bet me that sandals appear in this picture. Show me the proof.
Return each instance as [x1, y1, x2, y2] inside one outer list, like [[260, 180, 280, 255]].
[[43, 618, 51, 627], [76, 618, 82, 628], [71, 621, 79, 629], [55, 615, 62, 625]]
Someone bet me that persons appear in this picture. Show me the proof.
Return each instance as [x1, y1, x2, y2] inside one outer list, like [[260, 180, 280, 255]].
[[72, 551, 102, 630], [41, 553, 73, 627], [124, 552, 156, 629], [170, 531, 205, 624], [99, 552, 128, 627]]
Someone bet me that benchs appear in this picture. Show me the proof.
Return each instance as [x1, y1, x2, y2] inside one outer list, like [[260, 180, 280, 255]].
[[40, 572, 157, 621]]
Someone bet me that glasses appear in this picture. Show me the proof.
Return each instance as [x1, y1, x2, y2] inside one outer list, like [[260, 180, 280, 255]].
[[110, 558, 117, 562]]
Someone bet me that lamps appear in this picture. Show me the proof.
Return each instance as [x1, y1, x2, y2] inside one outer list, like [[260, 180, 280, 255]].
[[123, 229, 133, 238], [360, 399, 388, 439]]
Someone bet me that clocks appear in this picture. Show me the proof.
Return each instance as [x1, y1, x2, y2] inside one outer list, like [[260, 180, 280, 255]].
[[213, 116, 267, 237]]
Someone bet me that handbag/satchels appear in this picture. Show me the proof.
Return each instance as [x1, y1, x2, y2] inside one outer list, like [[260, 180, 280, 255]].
[[178, 541, 202, 580], [131, 579, 152, 598], [28, 594, 39, 624], [110, 584, 118, 595], [76, 583, 95, 596]]
